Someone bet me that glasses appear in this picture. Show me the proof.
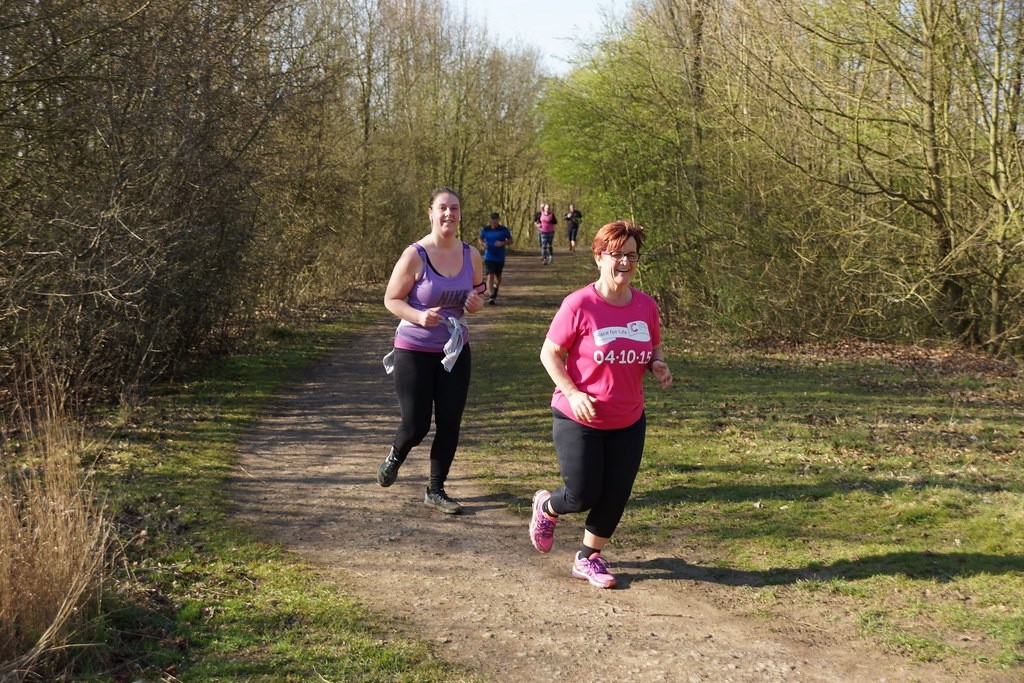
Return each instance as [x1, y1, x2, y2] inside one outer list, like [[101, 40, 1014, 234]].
[[601, 251, 641, 262]]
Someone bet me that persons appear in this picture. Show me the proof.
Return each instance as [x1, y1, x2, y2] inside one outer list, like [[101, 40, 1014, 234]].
[[533, 202, 557, 265], [529, 221, 672, 588], [479, 213, 513, 304], [376, 189, 485, 514], [562, 204, 582, 251]]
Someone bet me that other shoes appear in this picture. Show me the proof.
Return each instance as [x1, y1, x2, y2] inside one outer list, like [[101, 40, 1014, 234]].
[[549, 255, 552, 263], [542, 259, 547, 265], [489, 295, 494, 304], [493, 286, 498, 298]]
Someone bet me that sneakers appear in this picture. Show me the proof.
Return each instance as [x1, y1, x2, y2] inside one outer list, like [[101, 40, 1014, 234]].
[[424, 487, 462, 513], [528, 489, 558, 553], [377, 441, 407, 487], [572, 551, 618, 588]]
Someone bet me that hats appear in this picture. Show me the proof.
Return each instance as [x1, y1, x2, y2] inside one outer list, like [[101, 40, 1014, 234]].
[[491, 213, 499, 219]]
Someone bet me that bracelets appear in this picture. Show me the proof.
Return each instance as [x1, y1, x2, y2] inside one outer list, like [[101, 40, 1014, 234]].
[[650, 359, 664, 373]]
